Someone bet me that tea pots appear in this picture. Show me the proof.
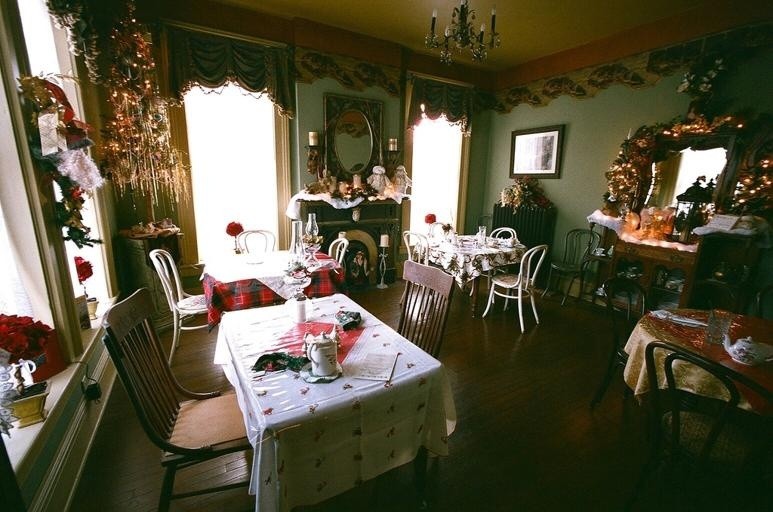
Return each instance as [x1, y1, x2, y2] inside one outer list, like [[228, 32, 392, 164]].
[[722, 333, 773, 367], [307, 330, 338, 376], [507, 237, 516, 248]]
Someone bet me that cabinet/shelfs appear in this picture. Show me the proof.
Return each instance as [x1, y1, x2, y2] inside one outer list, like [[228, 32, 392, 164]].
[[594, 217, 756, 317], [296, 195, 408, 282]]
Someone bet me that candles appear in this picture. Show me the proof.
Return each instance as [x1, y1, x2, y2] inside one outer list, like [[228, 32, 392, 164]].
[[390, 139, 398, 151], [309, 132, 317, 147], [379, 235, 389, 247]]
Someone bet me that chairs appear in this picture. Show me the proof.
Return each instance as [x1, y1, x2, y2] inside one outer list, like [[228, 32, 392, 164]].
[[645, 344, 773, 512], [589, 276, 657, 432], [429, 222, 456, 239], [469, 227, 518, 296], [541, 229, 600, 306], [238, 230, 275, 255], [398, 262, 455, 358], [104, 288, 277, 512], [686, 279, 737, 315], [400, 231, 446, 314], [329, 236, 349, 271], [483, 245, 549, 333], [149, 249, 220, 367]]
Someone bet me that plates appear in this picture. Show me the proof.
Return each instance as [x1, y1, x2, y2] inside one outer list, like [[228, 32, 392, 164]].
[[299, 361, 344, 384]]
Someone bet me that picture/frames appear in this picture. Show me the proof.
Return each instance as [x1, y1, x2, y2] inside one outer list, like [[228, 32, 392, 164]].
[[509, 125, 564, 180]]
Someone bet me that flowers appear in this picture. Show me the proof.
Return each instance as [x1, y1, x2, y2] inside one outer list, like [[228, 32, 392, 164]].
[[0, 314, 55, 393], [74, 256, 92, 297], [425, 214, 447, 233], [226, 222, 244, 248]]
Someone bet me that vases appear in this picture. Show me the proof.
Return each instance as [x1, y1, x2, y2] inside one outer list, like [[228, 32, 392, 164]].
[[442, 225, 451, 233], [232, 248, 244, 253], [84, 298, 99, 320], [7, 380, 54, 428]]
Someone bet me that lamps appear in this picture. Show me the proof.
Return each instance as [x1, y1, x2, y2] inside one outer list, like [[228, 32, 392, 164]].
[[424, 0, 502, 66]]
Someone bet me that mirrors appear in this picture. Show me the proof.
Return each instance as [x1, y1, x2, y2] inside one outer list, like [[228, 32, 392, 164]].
[[648, 136, 734, 233], [323, 94, 384, 181]]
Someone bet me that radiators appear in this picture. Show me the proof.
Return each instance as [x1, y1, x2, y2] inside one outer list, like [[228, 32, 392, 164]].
[[492, 203, 557, 289]]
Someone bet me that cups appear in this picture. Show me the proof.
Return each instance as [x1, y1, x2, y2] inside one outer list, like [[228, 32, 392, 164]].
[[479, 226, 486, 239], [705, 308, 732, 345]]
